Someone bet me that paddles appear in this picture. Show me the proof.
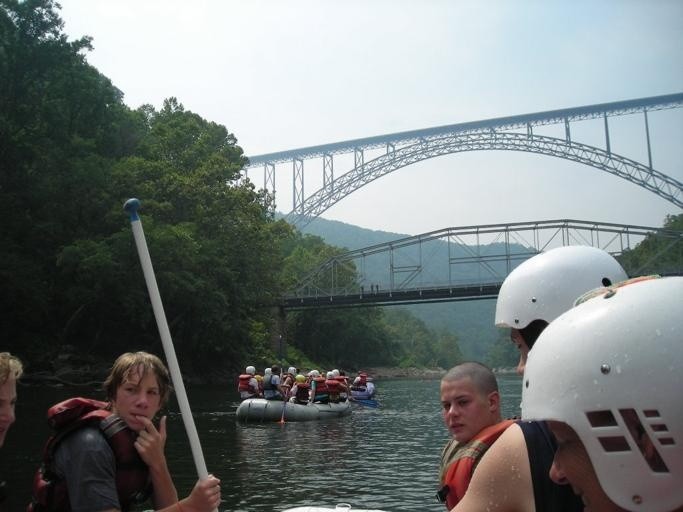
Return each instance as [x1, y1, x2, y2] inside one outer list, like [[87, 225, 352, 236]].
[[347, 399, 377, 408]]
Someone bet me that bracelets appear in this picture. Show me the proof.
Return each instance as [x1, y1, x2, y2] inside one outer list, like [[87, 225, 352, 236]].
[[174, 499, 184, 511]]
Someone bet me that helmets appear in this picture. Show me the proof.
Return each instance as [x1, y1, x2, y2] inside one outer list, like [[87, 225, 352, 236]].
[[244, 363, 341, 386], [492, 244, 629, 331]]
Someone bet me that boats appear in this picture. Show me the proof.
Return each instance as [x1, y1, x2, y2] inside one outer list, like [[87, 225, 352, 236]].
[[347, 382, 376, 401], [235, 396, 353, 425]]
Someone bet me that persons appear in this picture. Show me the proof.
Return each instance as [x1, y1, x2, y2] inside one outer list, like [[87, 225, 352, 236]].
[[235, 362, 367, 406], [24, 351, 222, 511], [1, 350, 24, 449], [447, 246, 628, 511], [435, 359, 517, 511]]
